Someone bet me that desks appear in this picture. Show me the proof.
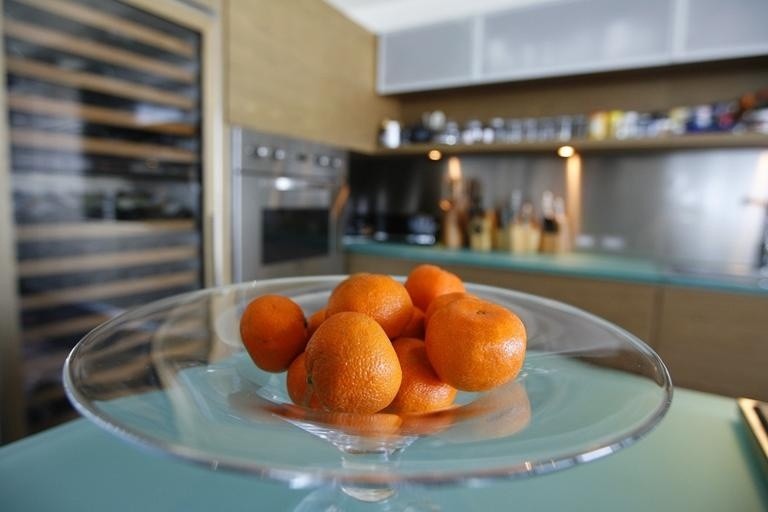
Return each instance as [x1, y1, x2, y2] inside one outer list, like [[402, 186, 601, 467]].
[[0, 311, 768, 512]]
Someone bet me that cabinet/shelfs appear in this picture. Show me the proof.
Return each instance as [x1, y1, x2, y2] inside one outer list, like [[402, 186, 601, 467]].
[[342, 239, 767, 400], [373, 2, 768, 103]]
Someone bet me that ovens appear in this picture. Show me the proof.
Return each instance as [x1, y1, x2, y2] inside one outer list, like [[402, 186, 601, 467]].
[[231, 125, 351, 284]]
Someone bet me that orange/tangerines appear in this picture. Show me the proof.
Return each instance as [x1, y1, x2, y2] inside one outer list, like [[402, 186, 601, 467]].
[[240, 264, 528, 424]]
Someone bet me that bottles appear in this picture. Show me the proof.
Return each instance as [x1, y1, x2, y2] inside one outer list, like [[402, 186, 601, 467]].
[[464, 178, 570, 257]]
